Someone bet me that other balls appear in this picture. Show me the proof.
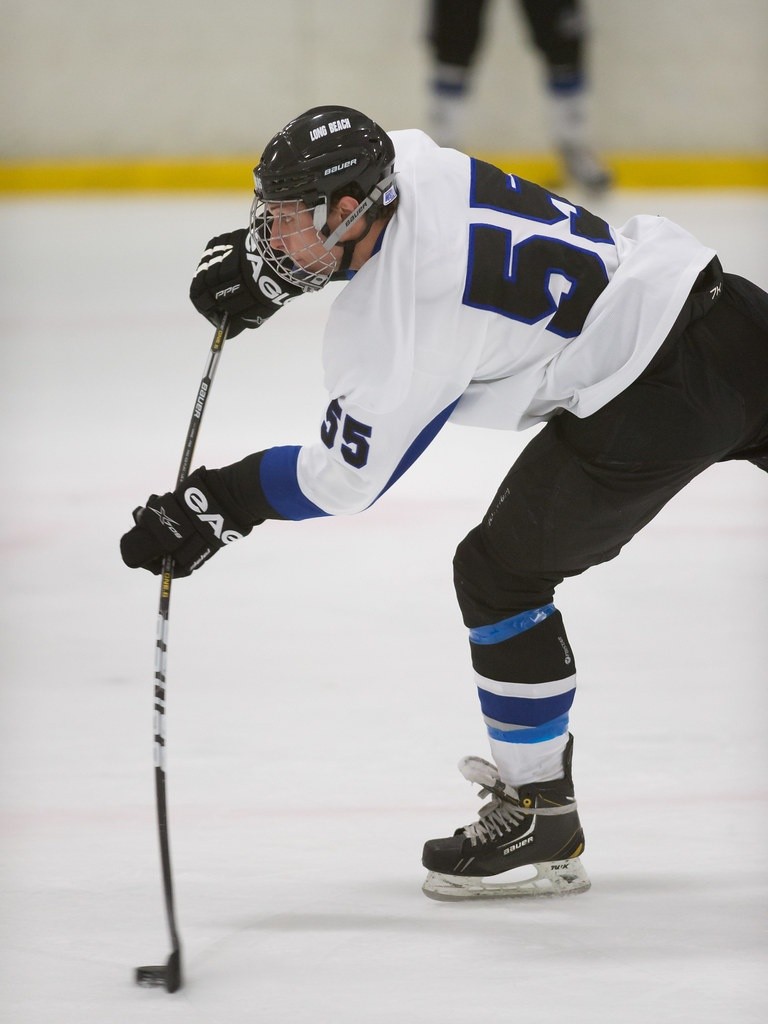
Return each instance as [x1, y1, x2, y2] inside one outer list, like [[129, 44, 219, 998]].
[[135, 966, 165, 982]]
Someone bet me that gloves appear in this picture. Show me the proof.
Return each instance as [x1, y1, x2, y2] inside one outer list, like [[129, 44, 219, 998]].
[[189, 213, 303, 339], [121, 466, 249, 578]]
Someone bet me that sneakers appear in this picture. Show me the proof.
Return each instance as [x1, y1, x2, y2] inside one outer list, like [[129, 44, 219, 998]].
[[422, 734, 593, 901], [552, 107, 617, 203], [426, 81, 470, 153]]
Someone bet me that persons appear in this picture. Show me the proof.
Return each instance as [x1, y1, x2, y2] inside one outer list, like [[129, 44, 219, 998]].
[[120, 105, 768, 898], [414, 0, 614, 198]]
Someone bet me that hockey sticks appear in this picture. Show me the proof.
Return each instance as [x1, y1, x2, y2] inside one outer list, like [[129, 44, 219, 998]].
[[152, 310, 232, 992]]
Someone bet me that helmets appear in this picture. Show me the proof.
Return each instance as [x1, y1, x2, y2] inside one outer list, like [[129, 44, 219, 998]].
[[254, 105, 397, 223]]
